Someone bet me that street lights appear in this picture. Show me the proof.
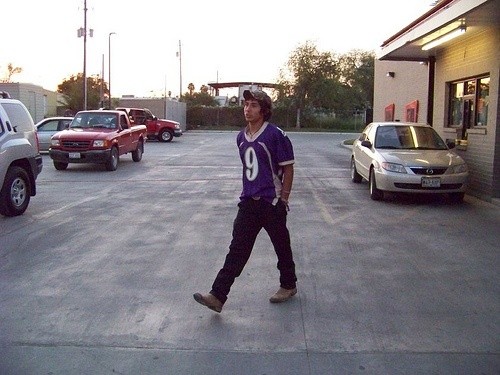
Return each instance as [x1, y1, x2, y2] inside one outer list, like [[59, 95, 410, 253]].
[[108, 32, 117, 110], [176, 40, 182, 100]]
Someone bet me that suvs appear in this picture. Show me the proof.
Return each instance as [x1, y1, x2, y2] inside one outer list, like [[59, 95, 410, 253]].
[[0, 90, 44, 218], [115, 107, 183, 143]]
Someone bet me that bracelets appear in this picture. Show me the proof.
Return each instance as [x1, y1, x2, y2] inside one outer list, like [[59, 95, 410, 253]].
[[283, 191, 290, 194]]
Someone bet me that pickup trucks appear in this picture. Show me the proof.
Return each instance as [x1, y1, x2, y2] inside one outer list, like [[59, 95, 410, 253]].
[[49, 109, 148, 172]]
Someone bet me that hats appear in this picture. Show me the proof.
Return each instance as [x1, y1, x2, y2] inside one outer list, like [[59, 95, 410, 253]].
[[243, 89, 271, 105]]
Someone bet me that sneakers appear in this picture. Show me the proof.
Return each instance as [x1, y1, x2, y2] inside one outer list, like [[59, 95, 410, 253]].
[[269, 287, 297, 303], [193, 293, 224, 313]]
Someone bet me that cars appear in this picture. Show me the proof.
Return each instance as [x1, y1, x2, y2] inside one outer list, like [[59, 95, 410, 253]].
[[35, 116, 74, 152], [349, 121, 469, 203]]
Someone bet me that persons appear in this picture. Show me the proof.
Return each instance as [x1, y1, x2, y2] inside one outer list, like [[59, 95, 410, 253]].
[[193, 89, 297, 313]]
